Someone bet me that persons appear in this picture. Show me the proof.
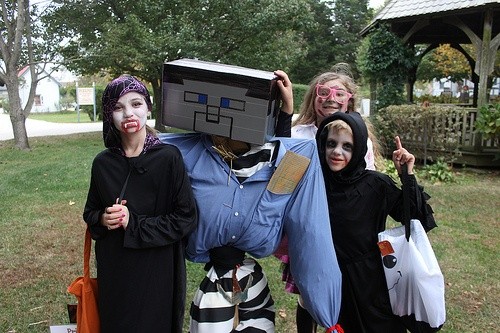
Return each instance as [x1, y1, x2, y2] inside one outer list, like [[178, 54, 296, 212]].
[[83, 76, 199, 333], [272, 70, 436, 333], [149, 58, 342, 333], [286, 72, 375, 333]]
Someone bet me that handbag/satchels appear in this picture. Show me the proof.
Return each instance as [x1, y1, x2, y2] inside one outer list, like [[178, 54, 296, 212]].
[[378, 221, 446, 333], [67, 225, 101, 333]]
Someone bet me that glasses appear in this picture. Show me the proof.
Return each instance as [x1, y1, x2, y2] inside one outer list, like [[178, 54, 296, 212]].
[[316, 83, 353, 104]]
